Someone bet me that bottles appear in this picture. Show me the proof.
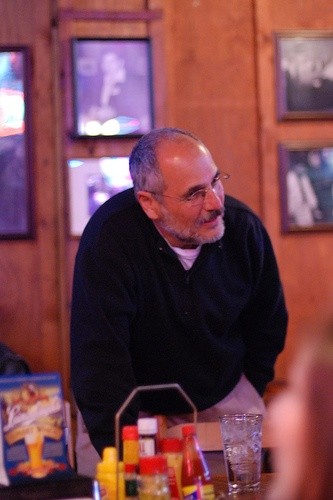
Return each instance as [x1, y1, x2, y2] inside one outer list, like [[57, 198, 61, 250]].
[[93, 415, 216, 500]]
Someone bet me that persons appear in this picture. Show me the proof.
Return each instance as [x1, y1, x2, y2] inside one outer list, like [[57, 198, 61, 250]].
[[285, 41, 333, 111], [286, 159, 322, 223], [306, 150, 333, 221], [84, 48, 146, 123], [69, 125, 288, 463]]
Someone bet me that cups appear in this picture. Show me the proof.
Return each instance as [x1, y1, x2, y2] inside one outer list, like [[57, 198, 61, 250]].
[[218, 414, 264, 494]]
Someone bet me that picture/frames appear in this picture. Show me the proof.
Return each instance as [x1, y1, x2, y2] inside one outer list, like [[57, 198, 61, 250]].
[[62, 155, 134, 239], [277, 140, 333, 234], [0, 45, 35, 243], [63, 38, 155, 139], [273, 30, 333, 120]]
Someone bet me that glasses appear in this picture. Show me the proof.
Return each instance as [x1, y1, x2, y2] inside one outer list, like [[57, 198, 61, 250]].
[[142, 169, 230, 205]]
